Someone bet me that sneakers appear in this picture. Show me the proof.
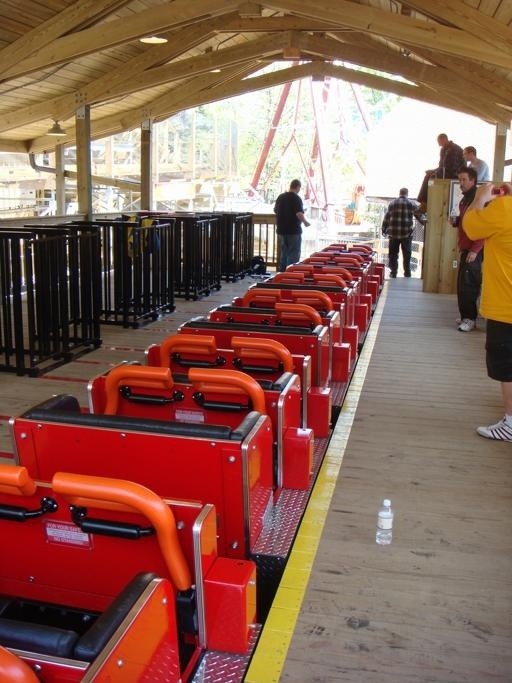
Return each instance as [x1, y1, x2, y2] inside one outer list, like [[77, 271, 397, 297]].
[[455, 318, 476, 331], [476, 416, 512, 444]]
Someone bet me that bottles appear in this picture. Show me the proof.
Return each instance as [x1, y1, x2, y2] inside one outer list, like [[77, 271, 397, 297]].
[[374, 499, 395, 545]]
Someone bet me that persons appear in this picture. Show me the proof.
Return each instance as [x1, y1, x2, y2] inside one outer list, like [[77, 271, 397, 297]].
[[461, 179, 512, 443], [462, 145, 491, 181], [448, 166, 484, 332], [415, 132, 468, 213], [380, 187, 427, 277], [274, 178, 311, 271]]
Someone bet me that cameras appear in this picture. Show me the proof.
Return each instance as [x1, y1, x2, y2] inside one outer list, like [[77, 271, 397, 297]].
[[491, 187, 505, 195], [450, 216, 459, 228]]
